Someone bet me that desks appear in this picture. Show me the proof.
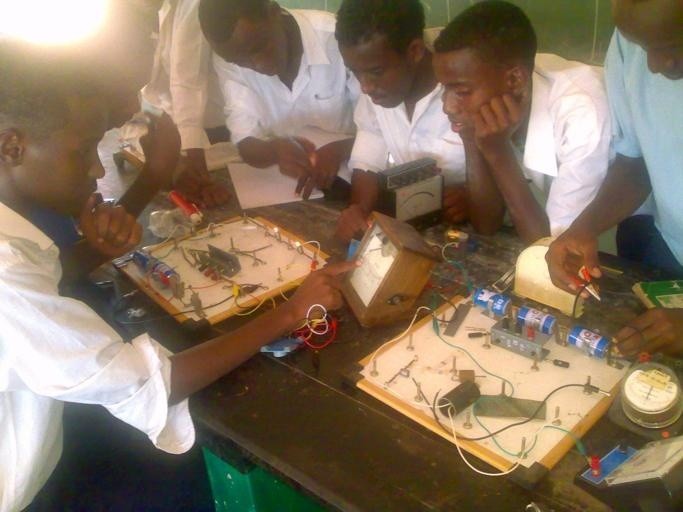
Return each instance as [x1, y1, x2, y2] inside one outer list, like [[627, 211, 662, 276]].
[[74, 125, 682, 512]]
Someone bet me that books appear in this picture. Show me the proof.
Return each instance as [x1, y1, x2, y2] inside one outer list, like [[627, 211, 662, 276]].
[[631, 274, 683, 318]]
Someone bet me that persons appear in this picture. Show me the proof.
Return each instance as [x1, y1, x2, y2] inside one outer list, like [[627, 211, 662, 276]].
[[433, 2, 630, 251], [333, 0, 473, 243], [1, 68, 365, 511], [197, 0, 358, 208], [28, 1, 180, 243], [543, 2, 682, 359]]
